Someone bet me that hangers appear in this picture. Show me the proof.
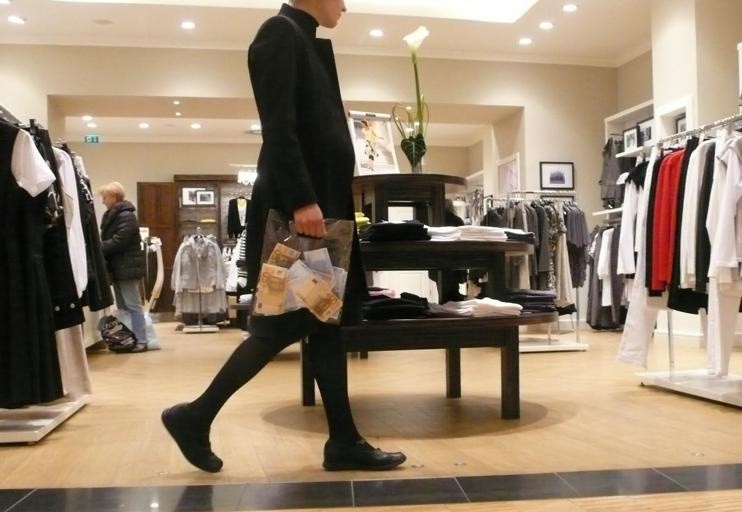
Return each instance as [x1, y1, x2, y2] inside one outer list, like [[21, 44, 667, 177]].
[[193, 228, 207, 241], [492, 196, 577, 207], [659, 113, 735, 146]]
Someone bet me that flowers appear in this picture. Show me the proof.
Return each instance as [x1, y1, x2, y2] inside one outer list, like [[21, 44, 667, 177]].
[[393, 26, 429, 169]]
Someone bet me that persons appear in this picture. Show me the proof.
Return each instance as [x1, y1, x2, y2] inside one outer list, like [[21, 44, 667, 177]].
[[97, 181, 148, 352], [161, 0, 406, 472], [361, 120, 384, 171]]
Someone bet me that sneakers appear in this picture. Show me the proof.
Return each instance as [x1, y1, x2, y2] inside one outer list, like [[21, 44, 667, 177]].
[[129, 343, 147, 352]]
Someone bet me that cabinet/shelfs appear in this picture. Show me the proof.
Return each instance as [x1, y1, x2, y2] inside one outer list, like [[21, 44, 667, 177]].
[[592, 146, 655, 216]]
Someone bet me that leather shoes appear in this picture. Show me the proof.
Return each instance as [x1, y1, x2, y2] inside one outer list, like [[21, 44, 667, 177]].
[[321, 439, 407, 471], [161, 402, 223, 473]]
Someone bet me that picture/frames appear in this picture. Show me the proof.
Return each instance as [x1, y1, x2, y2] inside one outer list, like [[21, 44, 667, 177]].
[[622, 125, 639, 151], [495, 152, 520, 194], [539, 161, 574, 189], [181, 186, 207, 206], [676, 116, 686, 133], [195, 190, 215, 206], [637, 116, 654, 146]]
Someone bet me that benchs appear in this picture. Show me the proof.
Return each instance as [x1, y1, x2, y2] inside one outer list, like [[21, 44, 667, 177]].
[[229, 304, 252, 333]]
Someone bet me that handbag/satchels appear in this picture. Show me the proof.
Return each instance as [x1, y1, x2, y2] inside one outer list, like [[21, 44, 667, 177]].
[[97, 315, 137, 352]]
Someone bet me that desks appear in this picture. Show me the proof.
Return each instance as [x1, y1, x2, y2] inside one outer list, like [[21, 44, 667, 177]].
[[301, 174, 559, 418]]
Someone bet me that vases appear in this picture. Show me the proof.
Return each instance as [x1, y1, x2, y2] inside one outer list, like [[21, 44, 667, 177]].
[[400, 119, 426, 174]]
